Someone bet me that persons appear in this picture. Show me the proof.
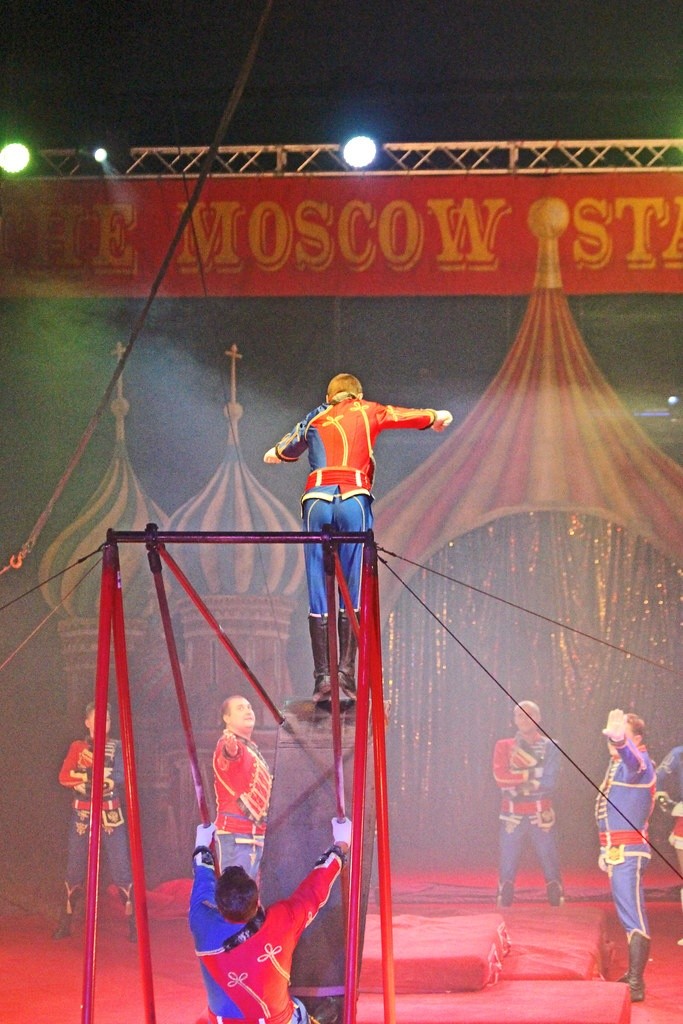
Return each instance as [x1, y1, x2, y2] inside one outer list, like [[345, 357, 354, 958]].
[[188, 817, 350, 1024], [657, 744, 683, 946], [263, 373, 454, 699], [212, 696, 273, 881], [492, 700, 564, 909], [50, 699, 138, 943], [593, 709, 657, 1002]]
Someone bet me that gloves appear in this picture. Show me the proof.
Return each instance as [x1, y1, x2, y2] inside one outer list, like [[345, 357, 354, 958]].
[[194, 821, 218, 850], [263, 445, 282, 464], [534, 767, 544, 778], [602, 709, 628, 742], [671, 800, 683, 817], [104, 767, 113, 779], [431, 409, 454, 433], [331, 816, 352, 850]]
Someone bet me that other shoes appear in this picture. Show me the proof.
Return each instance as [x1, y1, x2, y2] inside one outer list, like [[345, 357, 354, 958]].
[[677, 938, 683, 947]]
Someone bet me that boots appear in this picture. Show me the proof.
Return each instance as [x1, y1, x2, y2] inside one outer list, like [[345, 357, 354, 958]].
[[307, 611, 332, 702], [543, 875, 566, 908], [338, 607, 361, 699], [117, 884, 139, 943], [495, 875, 514, 909], [51, 880, 84, 941], [616, 929, 652, 1002]]
